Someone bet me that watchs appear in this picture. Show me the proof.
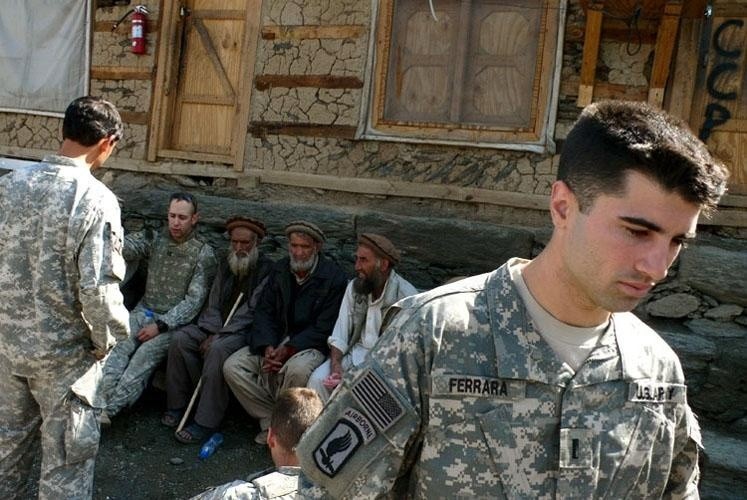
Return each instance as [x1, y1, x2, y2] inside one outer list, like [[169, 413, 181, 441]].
[[155, 319, 168, 334]]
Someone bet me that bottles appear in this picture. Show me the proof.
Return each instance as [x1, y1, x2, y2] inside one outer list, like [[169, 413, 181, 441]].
[[197, 432, 224, 460], [140, 311, 153, 328]]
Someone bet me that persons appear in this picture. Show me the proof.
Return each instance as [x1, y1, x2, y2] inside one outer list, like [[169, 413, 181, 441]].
[[222, 221, 348, 444], [294, 100, 731, 500], [0, 96, 130, 500], [306, 233, 418, 408], [99, 193, 217, 427], [188, 388, 325, 500], [161, 217, 277, 444]]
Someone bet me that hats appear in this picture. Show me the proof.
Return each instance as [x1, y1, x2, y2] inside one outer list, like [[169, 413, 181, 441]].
[[224, 215, 265, 239], [285, 220, 327, 242], [359, 233, 401, 265]]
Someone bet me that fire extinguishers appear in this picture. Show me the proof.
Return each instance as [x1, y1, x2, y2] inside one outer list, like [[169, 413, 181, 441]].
[[110, 5, 150, 54]]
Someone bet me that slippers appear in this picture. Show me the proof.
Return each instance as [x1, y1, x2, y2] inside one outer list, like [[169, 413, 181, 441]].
[[161, 408, 204, 443]]
